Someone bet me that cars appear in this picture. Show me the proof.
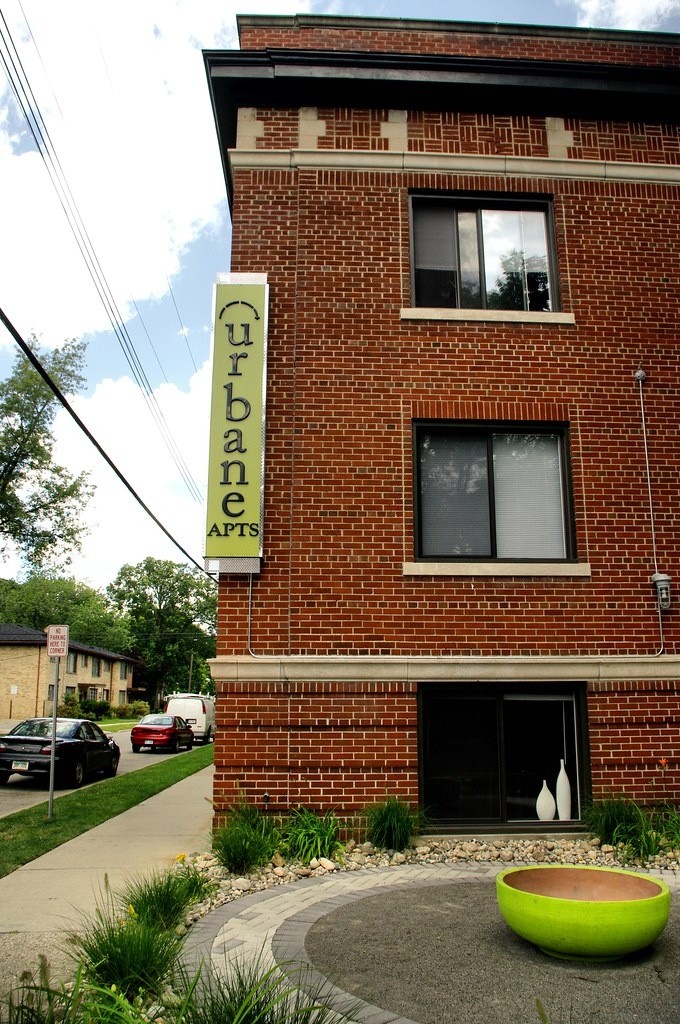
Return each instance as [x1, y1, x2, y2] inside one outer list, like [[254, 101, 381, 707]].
[[130, 714, 194, 753], [0, 718, 121, 785]]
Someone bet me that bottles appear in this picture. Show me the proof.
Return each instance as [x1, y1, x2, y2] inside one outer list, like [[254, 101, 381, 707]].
[[556, 759, 571, 820], [536, 780, 555, 820]]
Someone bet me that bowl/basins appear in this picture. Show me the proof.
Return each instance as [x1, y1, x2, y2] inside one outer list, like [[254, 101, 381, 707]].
[[496, 864, 672, 964]]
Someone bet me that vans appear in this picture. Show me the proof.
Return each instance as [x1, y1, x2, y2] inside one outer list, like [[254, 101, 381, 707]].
[[164, 693, 215, 743]]
